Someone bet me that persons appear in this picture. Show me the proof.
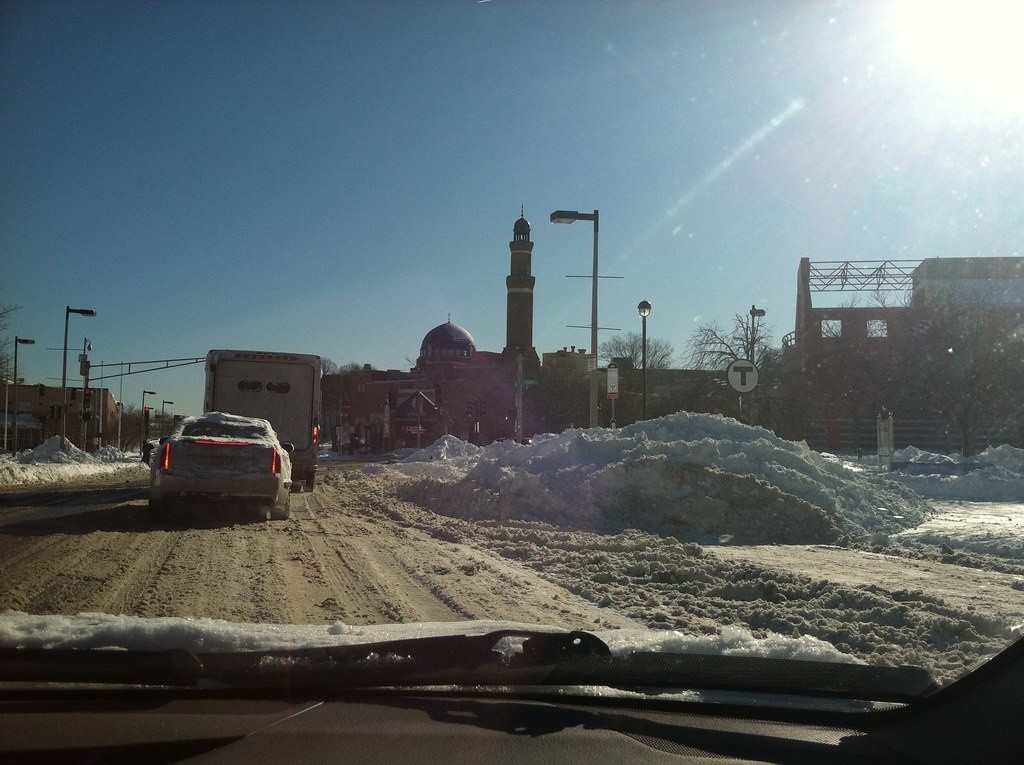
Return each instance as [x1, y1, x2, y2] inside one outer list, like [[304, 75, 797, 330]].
[[141, 437, 155, 465]]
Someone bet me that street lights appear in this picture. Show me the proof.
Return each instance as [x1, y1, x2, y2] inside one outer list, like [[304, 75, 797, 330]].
[[139, 390, 157, 456], [636, 299, 654, 422], [12, 335, 36, 458], [82, 336, 93, 451], [160, 400, 174, 437], [548, 208, 626, 430], [59, 306, 99, 451]]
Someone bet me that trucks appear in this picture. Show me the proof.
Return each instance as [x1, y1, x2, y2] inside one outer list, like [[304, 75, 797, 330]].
[[200, 347, 324, 494]]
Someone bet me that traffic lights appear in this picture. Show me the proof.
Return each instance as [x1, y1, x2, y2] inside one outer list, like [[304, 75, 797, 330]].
[[479, 399, 488, 417], [465, 399, 475, 418], [33, 384, 39, 397], [82, 387, 92, 408], [84, 413, 91, 421], [48, 404, 56, 420], [39, 383, 46, 396], [70, 388, 77, 400], [55, 404, 62, 419]]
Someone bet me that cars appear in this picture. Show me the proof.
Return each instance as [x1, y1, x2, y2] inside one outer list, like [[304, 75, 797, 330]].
[[145, 411, 296, 522]]
